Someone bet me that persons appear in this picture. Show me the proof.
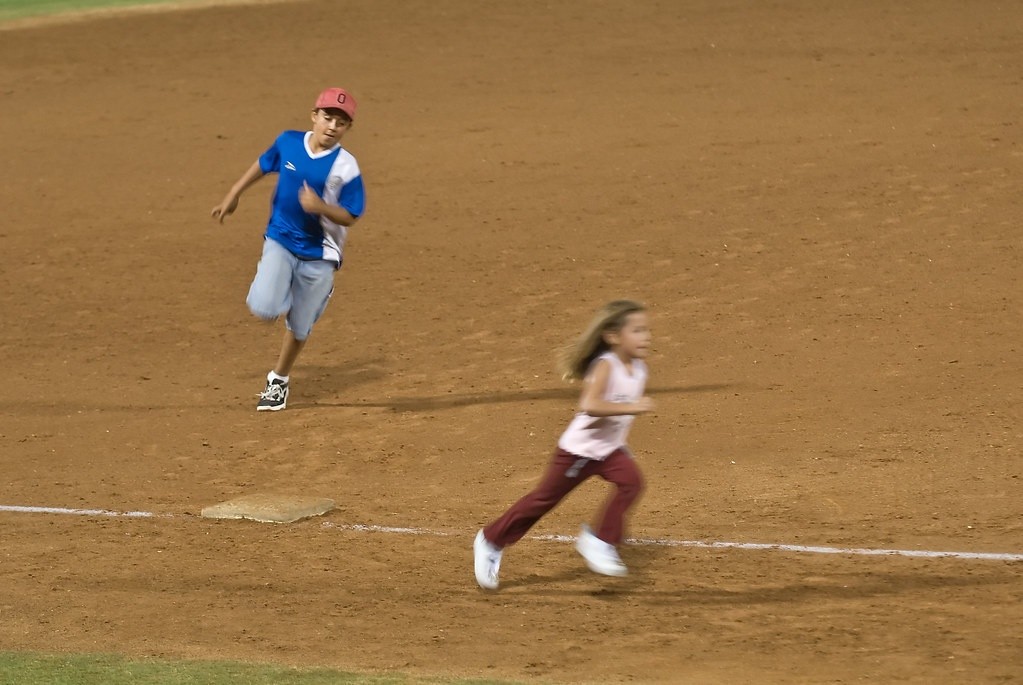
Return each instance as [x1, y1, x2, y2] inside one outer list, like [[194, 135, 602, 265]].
[[472, 301, 655, 593], [211, 88, 366, 411]]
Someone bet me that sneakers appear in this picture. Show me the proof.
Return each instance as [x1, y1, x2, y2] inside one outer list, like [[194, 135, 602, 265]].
[[256, 377, 288, 411]]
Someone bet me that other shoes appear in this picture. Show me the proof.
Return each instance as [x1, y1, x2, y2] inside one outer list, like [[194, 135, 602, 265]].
[[474, 527, 501, 589], [575, 531, 628, 577]]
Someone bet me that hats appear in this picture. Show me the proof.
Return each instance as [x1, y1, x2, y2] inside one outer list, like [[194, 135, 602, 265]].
[[311, 88, 357, 122]]
